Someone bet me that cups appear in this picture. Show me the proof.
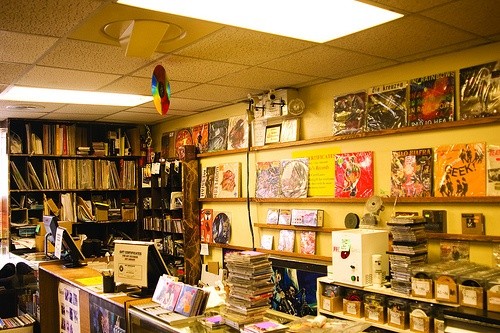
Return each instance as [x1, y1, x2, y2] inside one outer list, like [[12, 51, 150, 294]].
[[103, 276, 115, 293]]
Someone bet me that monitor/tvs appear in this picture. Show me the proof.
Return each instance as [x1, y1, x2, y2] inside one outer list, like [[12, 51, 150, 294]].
[[42, 215, 88, 268], [113, 239, 170, 298]]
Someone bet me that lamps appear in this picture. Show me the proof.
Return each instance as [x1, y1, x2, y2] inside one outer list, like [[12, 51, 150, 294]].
[[247, 88, 305, 123]]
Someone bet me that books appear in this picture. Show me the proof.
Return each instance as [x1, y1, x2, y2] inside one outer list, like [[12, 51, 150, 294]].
[[0, 270, 40, 330], [10, 123, 138, 249], [142, 60, 500, 333]]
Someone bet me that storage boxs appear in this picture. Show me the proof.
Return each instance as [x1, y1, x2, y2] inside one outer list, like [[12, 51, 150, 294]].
[[94, 208, 108, 221], [434, 314, 450, 333], [409, 308, 436, 333], [342, 298, 365, 319], [458, 279, 488, 310], [411, 272, 435, 299], [387, 306, 412, 330], [433, 275, 460, 304], [122, 207, 135, 220], [320, 293, 344, 313], [364, 303, 389, 324], [486, 284, 500, 313], [36, 221, 72, 236], [35, 234, 44, 252]]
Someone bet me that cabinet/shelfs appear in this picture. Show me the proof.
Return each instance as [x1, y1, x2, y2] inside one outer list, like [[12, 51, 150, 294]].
[[0, 114, 500, 333]]
